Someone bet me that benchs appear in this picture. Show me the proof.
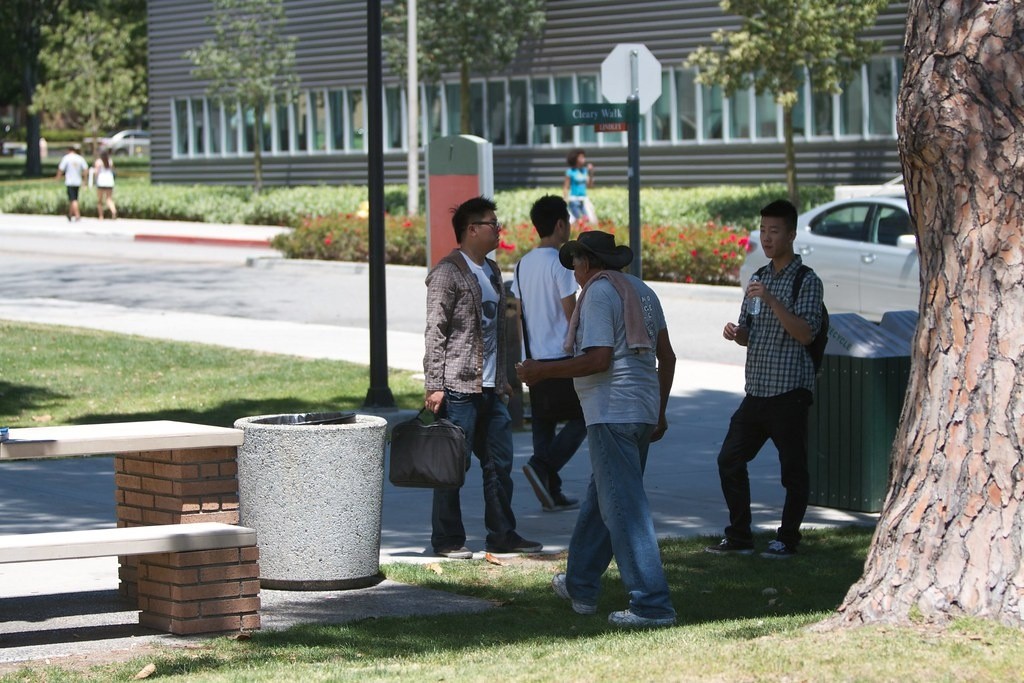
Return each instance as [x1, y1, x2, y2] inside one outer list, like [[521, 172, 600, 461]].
[[820, 222, 863, 240], [0, 521, 262, 635]]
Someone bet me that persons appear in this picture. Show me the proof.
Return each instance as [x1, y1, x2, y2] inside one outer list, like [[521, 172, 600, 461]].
[[423, 196, 543, 559], [706, 199, 824, 556], [517, 230, 677, 624], [94, 152, 116, 220], [56, 147, 89, 222], [511, 195, 587, 511], [564, 149, 593, 226]]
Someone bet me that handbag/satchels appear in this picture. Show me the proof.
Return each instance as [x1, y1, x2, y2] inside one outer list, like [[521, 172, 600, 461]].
[[530, 374, 583, 421], [389, 406, 465, 489]]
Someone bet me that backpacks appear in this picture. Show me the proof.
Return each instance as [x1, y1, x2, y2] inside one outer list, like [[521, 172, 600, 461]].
[[745, 265, 829, 376]]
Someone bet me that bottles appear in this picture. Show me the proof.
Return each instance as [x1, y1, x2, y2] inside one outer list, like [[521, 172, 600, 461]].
[[746, 275, 762, 315]]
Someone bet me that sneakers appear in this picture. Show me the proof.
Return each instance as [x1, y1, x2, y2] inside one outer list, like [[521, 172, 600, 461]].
[[522, 457, 555, 510], [436, 544, 472, 558], [543, 494, 580, 512], [486, 536, 543, 553], [552, 573, 597, 615], [607, 609, 676, 625], [706, 539, 754, 554], [760, 540, 796, 559]]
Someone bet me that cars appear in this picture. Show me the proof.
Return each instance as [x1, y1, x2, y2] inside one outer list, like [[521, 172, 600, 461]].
[[97, 128, 150, 160], [737, 185, 921, 326]]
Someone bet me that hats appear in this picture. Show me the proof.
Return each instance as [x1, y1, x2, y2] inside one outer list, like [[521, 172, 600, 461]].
[[559, 231, 633, 270]]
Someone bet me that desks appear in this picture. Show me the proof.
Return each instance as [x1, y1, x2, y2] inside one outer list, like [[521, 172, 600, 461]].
[[0, 421, 244, 599]]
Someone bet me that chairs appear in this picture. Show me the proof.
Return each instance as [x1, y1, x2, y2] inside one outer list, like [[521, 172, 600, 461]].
[[879, 217, 909, 246]]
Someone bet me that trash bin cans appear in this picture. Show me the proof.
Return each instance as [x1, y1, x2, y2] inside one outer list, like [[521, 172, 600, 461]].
[[503, 296, 526, 425], [233, 410, 387, 591], [802, 353, 912, 513]]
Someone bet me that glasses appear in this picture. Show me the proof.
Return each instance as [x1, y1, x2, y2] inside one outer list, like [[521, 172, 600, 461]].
[[471, 221, 502, 231]]
